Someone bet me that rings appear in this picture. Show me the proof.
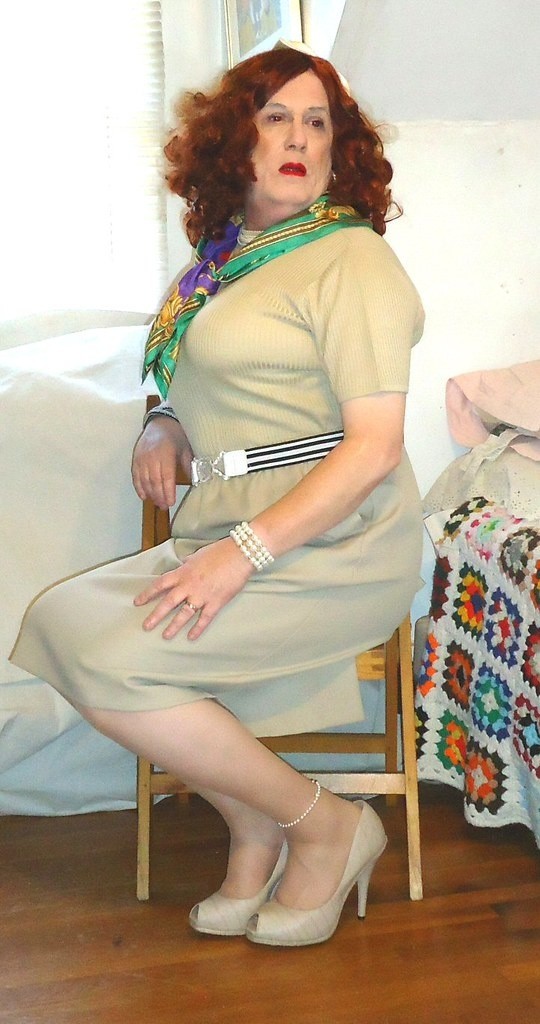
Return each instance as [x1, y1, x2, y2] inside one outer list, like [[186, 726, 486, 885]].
[[184, 601, 198, 613]]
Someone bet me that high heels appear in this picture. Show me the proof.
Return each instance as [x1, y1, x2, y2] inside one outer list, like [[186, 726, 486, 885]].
[[187, 836, 288, 936], [245, 799, 389, 947]]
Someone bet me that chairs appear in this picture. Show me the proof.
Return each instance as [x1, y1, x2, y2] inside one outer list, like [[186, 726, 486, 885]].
[[134, 394, 426, 902]]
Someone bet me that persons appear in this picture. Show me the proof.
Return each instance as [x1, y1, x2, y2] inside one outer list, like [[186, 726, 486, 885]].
[[10, 40, 427, 948]]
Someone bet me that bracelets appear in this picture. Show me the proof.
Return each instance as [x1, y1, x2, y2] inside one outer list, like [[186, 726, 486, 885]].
[[229, 521, 274, 573]]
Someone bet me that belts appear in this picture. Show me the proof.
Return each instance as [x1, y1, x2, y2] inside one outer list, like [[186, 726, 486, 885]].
[[191, 429, 346, 487]]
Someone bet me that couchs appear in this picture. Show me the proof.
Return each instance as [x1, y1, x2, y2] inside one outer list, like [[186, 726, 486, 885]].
[[422, 355, 540, 849]]
